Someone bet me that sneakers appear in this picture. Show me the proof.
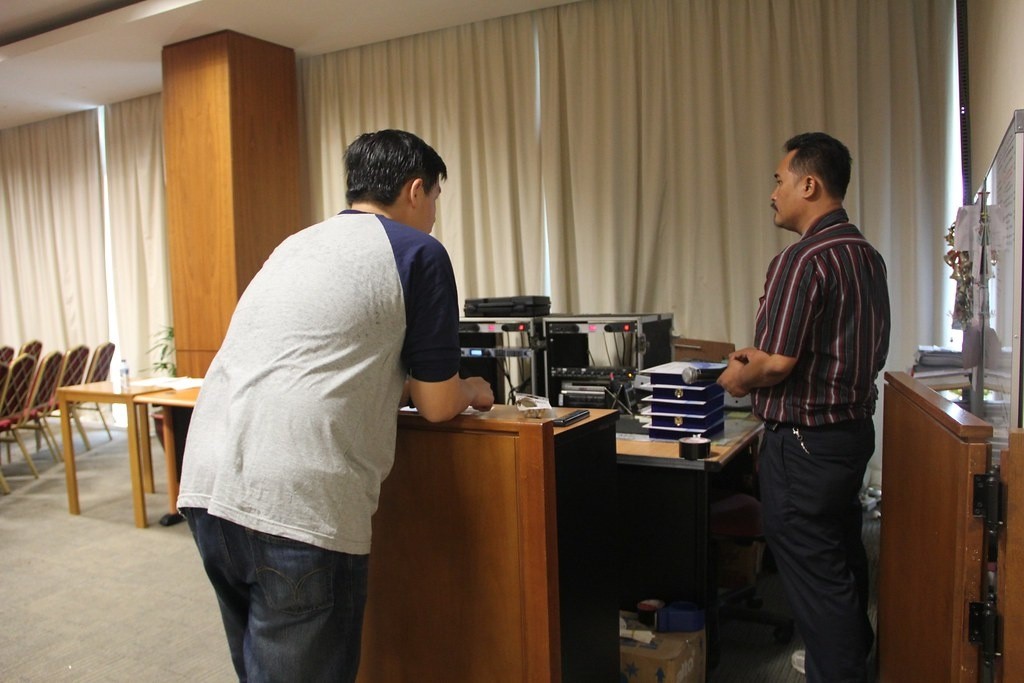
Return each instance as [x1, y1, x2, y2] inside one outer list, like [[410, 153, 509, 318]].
[[790, 649, 806, 675]]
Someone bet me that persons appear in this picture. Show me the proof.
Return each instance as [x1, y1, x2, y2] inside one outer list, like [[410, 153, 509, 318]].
[[177, 130, 494, 683], [717, 132, 891, 683]]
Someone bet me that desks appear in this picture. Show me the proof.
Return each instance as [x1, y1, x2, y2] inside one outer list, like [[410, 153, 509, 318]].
[[352, 401, 619, 681], [133, 386, 203, 526], [56, 378, 173, 529], [616, 407, 767, 632]]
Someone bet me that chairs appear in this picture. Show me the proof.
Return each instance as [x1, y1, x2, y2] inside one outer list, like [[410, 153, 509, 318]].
[[0, 340, 115, 496]]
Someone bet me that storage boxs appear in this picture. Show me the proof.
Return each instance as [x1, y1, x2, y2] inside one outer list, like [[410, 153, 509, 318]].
[[619, 611, 706, 683]]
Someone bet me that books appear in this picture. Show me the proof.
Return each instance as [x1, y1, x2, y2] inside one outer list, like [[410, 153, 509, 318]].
[[911, 346, 971, 390]]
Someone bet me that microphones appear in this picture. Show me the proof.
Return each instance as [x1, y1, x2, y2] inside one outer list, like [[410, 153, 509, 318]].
[[682, 367, 727, 383]]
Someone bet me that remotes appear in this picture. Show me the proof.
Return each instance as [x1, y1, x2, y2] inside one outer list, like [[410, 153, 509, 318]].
[[551, 409, 589, 427]]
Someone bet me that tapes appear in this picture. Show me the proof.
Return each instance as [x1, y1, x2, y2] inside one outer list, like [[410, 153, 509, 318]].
[[636, 597, 666, 625], [678, 436, 711, 460]]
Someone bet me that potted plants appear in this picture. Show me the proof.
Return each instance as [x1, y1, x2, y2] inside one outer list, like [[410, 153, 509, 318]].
[[145, 326, 177, 451]]
